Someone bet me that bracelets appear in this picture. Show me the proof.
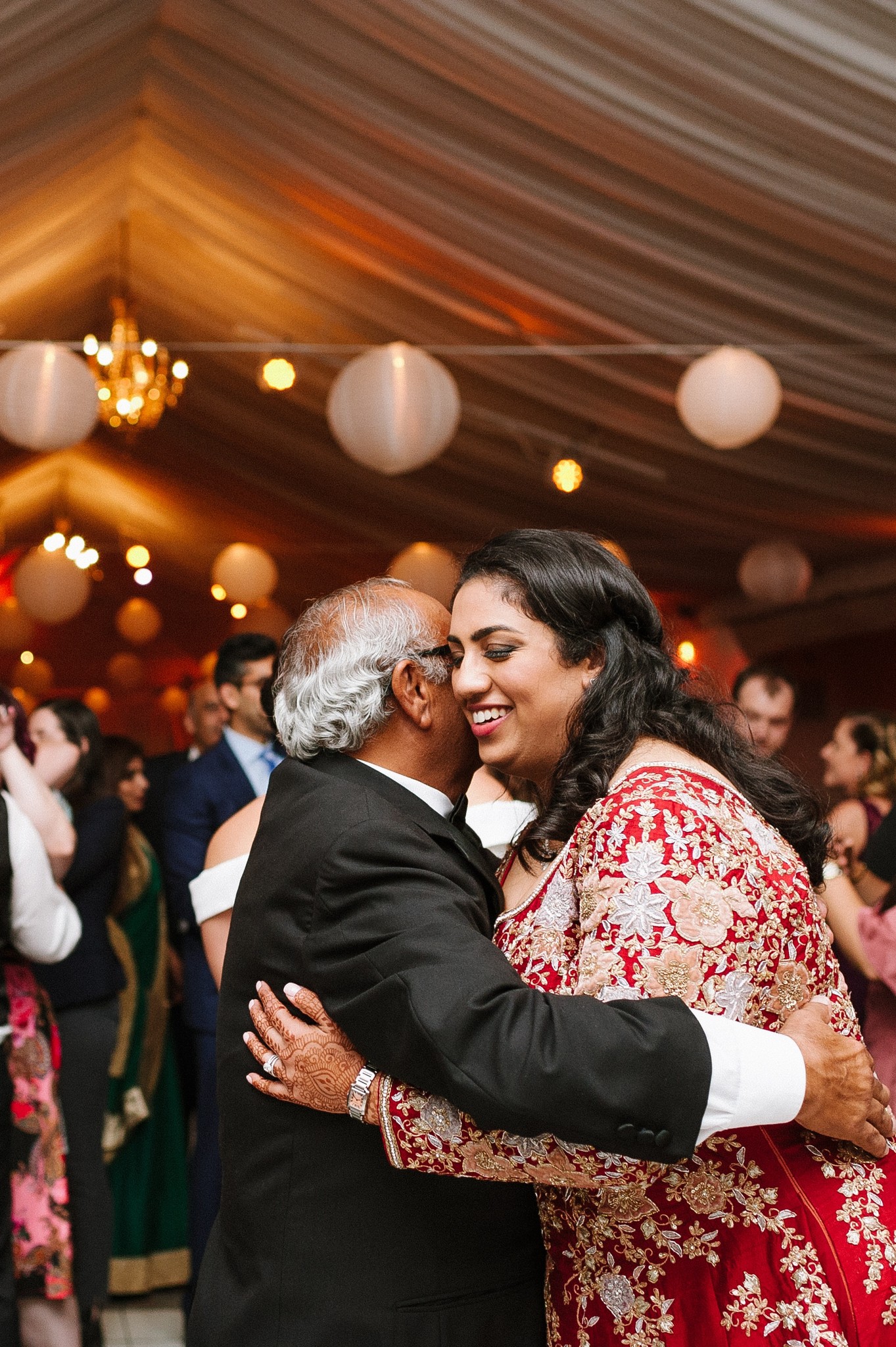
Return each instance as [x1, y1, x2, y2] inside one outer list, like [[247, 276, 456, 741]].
[[848, 859, 870, 885]]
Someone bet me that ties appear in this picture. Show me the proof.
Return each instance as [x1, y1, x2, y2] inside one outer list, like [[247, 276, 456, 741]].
[[260, 748, 276, 775]]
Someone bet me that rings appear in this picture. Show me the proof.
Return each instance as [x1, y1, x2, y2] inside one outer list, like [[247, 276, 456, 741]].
[[261, 1053, 279, 1078]]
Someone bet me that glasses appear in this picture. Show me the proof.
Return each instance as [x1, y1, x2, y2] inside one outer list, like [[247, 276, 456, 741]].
[[240, 675, 269, 691]]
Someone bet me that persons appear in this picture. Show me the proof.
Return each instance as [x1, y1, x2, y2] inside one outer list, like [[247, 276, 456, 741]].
[[185, 760, 548, 997], [723, 657, 802, 764], [129, 674, 235, 875], [89, 734, 195, 1299], [817, 711, 896, 861], [240, 526, 896, 1347], [0, 686, 81, 1347], [809, 856, 896, 1127], [186, 574, 895, 1346], [825, 793, 896, 906], [0, 786, 87, 1347], [165, 626, 292, 1342], [25, 695, 132, 1347]]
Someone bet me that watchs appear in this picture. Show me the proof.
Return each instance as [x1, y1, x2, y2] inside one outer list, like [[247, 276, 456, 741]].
[[345, 1059, 381, 1126], [819, 860, 844, 880]]
